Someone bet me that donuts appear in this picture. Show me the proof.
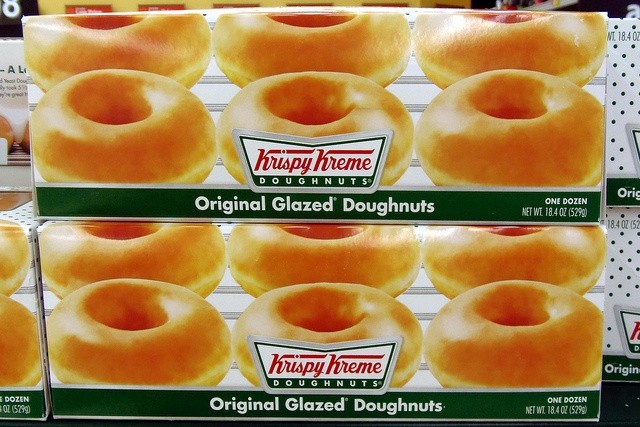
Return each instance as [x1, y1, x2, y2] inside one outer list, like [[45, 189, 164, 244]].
[[30, 70, 217, 185], [215, 71, 416, 185], [412, 11, 606, 88], [416, 67, 605, 188], [229, 283, 422, 387], [421, 220, 605, 300], [229, 223, 421, 298], [213, 8, 412, 95], [423, 278, 602, 390], [37, 222, 228, 299], [47, 277, 231, 385], [0, 291, 48, 387], [0, 219, 30, 298], [24, 13, 212, 95], [0, 193, 32, 210]]
